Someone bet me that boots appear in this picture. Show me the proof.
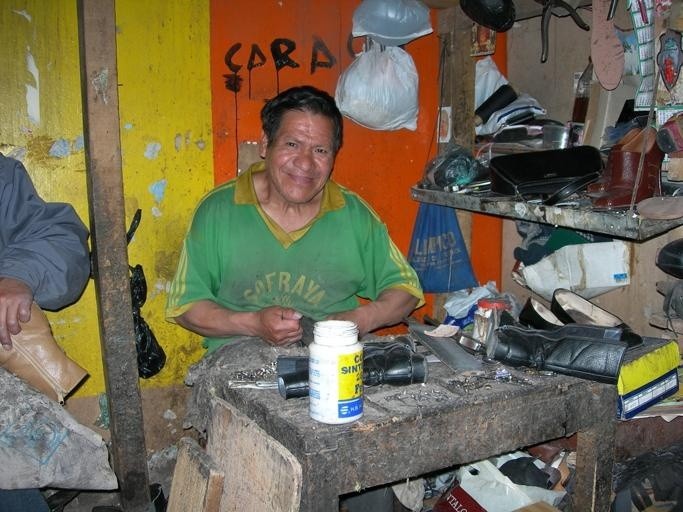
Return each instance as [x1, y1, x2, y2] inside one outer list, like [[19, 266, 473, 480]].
[[485, 323, 628, 384], [0, 298, 89, 405], [272, 333, 429, 400]]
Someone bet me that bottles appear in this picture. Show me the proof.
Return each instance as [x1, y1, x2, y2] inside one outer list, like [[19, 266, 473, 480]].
[[309, 320, 363, 424], [473, 297, 505, 348]]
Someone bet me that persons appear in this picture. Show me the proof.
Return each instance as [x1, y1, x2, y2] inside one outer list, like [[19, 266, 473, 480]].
[[0, 153, 91, 351], [164, 86, 426, 358]]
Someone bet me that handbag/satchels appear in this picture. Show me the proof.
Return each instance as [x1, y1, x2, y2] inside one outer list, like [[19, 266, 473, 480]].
[[485, 141, 605, 205]]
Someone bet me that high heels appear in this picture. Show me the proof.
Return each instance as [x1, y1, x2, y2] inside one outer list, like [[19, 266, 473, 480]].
[[591, 125, 666, 212]]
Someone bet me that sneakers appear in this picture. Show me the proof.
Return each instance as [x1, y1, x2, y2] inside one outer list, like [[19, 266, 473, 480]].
[[654, 236, 682, 322]]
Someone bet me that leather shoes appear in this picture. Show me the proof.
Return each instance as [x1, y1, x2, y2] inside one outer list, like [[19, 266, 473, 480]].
[[518, 296, 565, 331], [549, 287, 643, 351]]
